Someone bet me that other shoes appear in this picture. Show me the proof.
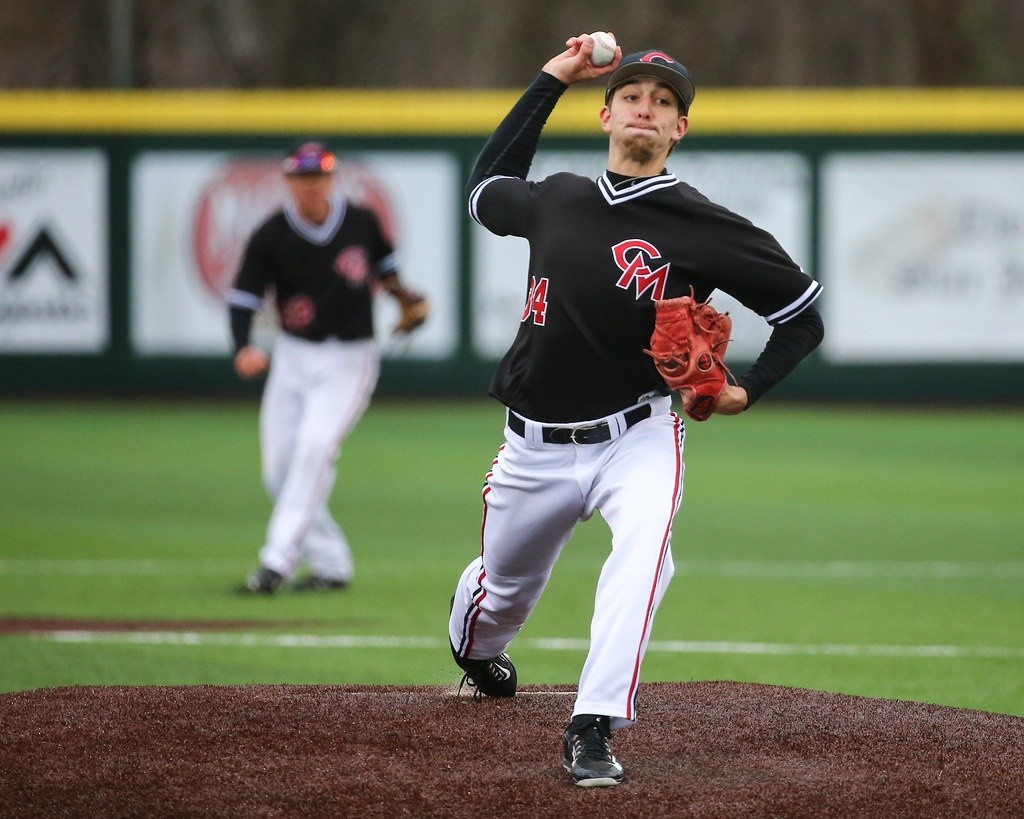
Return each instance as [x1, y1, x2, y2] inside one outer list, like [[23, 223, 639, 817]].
[[285, 571, 342, 591], [235, 563, 284, 594]]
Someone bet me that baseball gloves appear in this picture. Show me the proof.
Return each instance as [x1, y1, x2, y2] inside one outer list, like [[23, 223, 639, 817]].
[[649, 295, 732, 423]]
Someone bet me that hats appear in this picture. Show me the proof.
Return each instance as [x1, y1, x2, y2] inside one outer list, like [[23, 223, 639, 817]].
[[605, 50, 695, 110], [279, 144, 339, 177]]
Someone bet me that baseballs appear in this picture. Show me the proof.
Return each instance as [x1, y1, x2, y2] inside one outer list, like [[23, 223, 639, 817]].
[[587, 31, 617, 67]]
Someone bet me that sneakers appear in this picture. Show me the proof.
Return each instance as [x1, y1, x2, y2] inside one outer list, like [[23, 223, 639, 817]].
[[561, 720, 623, 786], [449, 595, 517, 697]]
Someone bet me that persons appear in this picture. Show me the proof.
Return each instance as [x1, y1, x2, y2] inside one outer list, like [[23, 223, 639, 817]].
[[226, 141, 428, 598], [446, 30, 825, 785]]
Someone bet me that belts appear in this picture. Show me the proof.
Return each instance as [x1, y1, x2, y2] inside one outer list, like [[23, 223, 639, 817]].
[[508, 403, 651, 444]]
